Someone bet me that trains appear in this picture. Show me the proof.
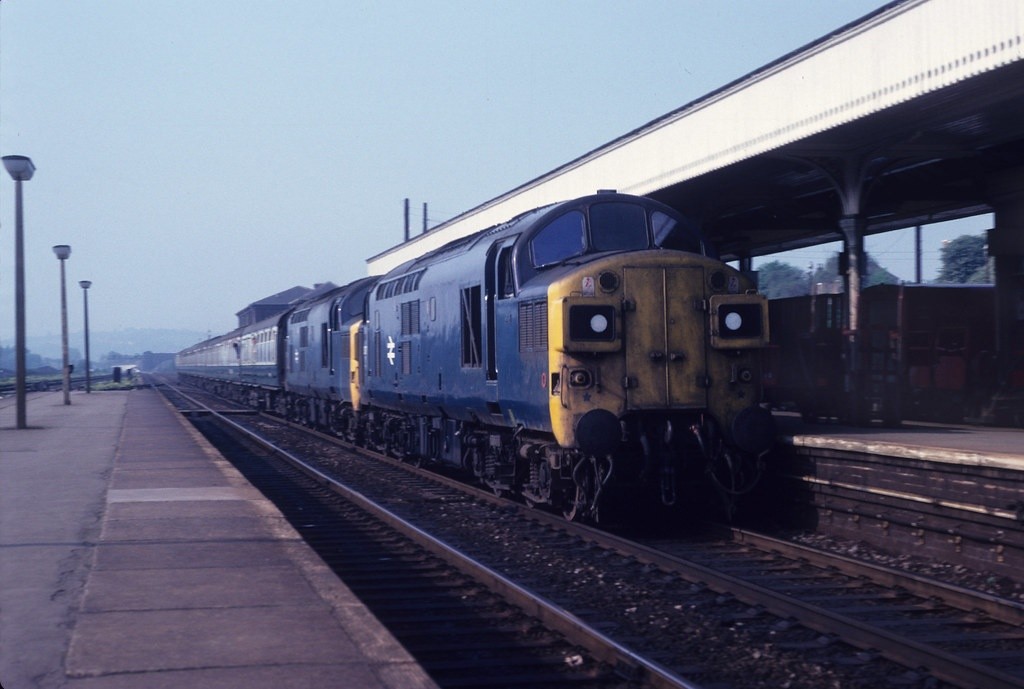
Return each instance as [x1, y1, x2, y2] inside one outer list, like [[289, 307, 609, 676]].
[[173, 188, 775, 531]]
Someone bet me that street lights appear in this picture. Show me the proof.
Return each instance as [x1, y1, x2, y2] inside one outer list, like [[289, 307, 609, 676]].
[[78, 280, 92, 394], [53, 245, 71, 405], [1, 155, 37, 429]]
